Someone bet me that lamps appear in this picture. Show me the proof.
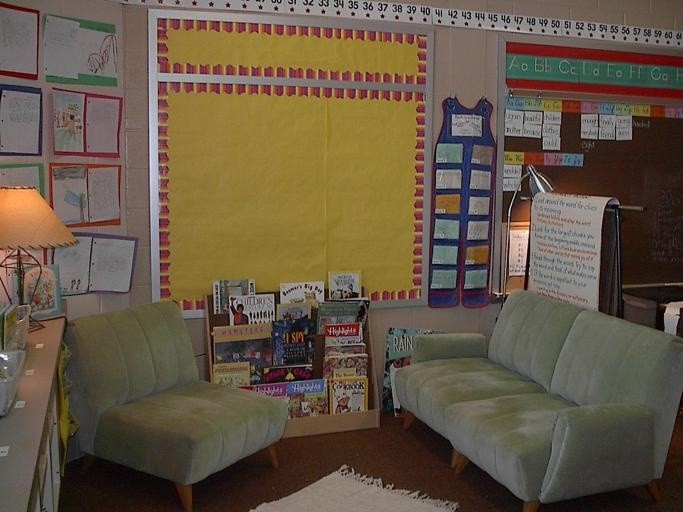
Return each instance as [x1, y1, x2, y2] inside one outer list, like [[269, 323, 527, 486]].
[[494, 163, 554, 310], [0, 183, 77, 329]]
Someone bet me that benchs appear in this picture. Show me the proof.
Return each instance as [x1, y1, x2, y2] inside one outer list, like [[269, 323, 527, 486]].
[[396, 285, 682, 512]]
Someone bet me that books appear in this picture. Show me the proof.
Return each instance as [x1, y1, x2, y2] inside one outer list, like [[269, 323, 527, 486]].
[[0, 304, 17, 352], [213, 270, 370, 418]]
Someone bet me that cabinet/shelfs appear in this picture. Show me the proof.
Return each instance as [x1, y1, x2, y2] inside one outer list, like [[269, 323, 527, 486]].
[[203, 290, 381, 440], [0, 298, 85, 512]]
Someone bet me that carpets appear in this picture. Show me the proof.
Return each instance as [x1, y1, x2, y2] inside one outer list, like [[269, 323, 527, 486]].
[[247, 466, 459, 512]]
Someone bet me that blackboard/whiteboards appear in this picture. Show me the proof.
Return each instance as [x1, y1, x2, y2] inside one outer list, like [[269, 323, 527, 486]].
[[491, 31, 683, 302]]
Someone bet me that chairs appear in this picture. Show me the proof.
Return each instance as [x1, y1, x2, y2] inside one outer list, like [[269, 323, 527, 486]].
[[62, 297, 290, 512]]
